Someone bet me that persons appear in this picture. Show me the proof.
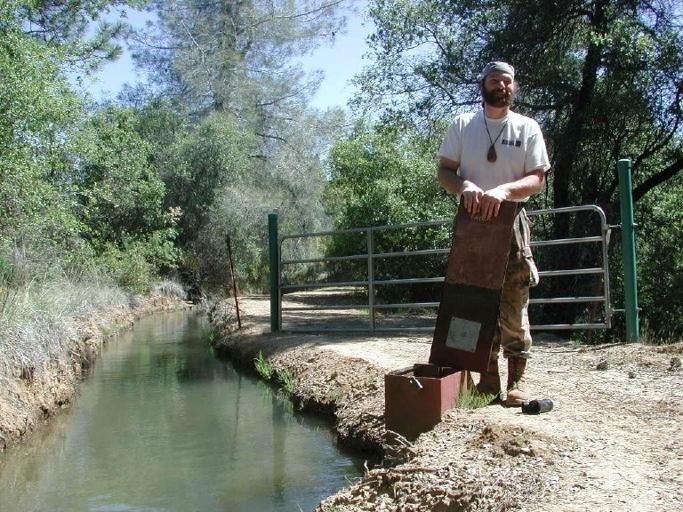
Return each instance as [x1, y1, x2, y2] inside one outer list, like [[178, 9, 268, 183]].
[[437, 61, 551, 406]]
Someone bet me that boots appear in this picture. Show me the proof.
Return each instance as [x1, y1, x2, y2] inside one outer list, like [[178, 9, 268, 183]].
[[471, 357, 502, 396], [504, 356, 531, 407]]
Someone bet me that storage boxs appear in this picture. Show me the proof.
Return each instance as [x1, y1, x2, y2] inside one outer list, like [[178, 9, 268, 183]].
[[384, 363, 469, 440]]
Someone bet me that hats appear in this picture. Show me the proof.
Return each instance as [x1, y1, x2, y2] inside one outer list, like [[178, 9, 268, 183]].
[[480, 59, 517, 82]]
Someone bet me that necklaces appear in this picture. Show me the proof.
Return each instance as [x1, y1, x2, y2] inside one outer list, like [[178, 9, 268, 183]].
[[483, 110, 509, 162]]
